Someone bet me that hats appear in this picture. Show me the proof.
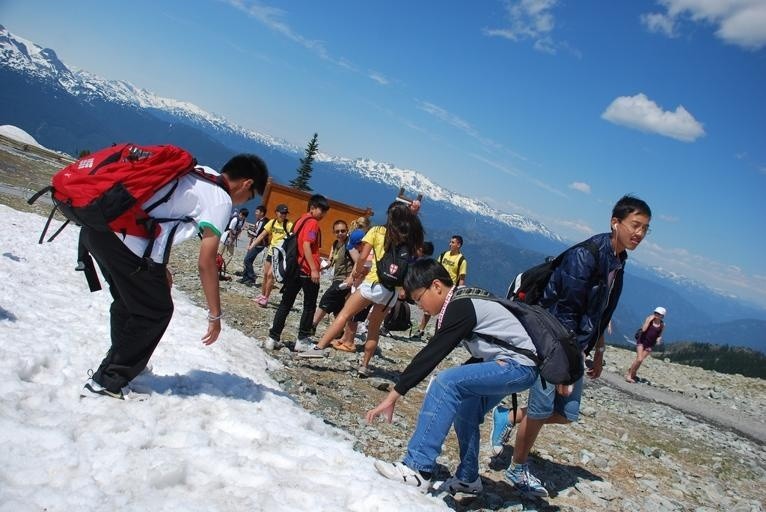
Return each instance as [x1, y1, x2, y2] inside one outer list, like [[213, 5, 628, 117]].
[[653, 306, 666, 317], [277, 204, 289, 214], [347, 229, 365, 250]]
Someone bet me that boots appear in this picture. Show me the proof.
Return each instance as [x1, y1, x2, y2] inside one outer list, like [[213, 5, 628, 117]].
[[503, 456, 549, 497], [490, 404, 515, 458]]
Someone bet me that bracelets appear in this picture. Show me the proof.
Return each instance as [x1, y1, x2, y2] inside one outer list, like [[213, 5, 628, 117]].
[[206, 311, 225, 322], [595, 346, 605, 353]]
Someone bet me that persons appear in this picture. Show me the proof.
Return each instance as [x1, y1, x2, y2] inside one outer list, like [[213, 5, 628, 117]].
[[489, 194, 653, 497], [364, 255, 540, 495], [624, 306, 667, 383], [78, 152, 270, 400], [601, 320, 612, 366]]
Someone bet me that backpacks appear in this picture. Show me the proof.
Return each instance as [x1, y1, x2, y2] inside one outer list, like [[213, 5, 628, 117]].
[[272, 217, 319, 289], [505, 240, 605, 305], [51, 143, 230, 240], [447, 288, 584, 385], [373, 224, 411, 286], [384, 299, 410, 331]]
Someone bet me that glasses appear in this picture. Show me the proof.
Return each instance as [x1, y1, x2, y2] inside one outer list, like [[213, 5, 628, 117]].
[[248, 187, 256, 201], [414, 281, 432, 307], [616, 217, 652, 236]]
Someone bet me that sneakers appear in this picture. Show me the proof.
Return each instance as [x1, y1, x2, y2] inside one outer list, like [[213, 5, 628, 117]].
[[294, 326, 357, 358], [80, 377, 151, 402], [410, 330, 424, 341], [431, 474, 484, 494], [237, 278, 255, 287], [358, 366, 369, 376], [373, 459, 432, 493], [251, 296, 268, 306], [266, 336, 288, 350], [356, 320, 392, 337]]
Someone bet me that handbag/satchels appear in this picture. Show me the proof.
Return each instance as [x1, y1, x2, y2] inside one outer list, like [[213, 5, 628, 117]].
[[216, 253, 227, 273], [635, 329, 645, 340]]
